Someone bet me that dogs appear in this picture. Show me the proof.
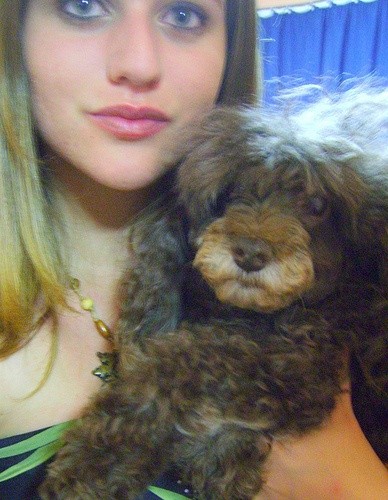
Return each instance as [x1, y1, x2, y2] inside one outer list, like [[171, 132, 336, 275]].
[[39, 102, 388, 500]]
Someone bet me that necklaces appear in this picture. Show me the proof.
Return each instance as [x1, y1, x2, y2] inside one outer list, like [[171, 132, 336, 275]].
[[55, 251, 120, 382]]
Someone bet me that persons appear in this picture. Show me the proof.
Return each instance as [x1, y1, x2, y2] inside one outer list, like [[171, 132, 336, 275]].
[[0, 0, 388, 500]]
